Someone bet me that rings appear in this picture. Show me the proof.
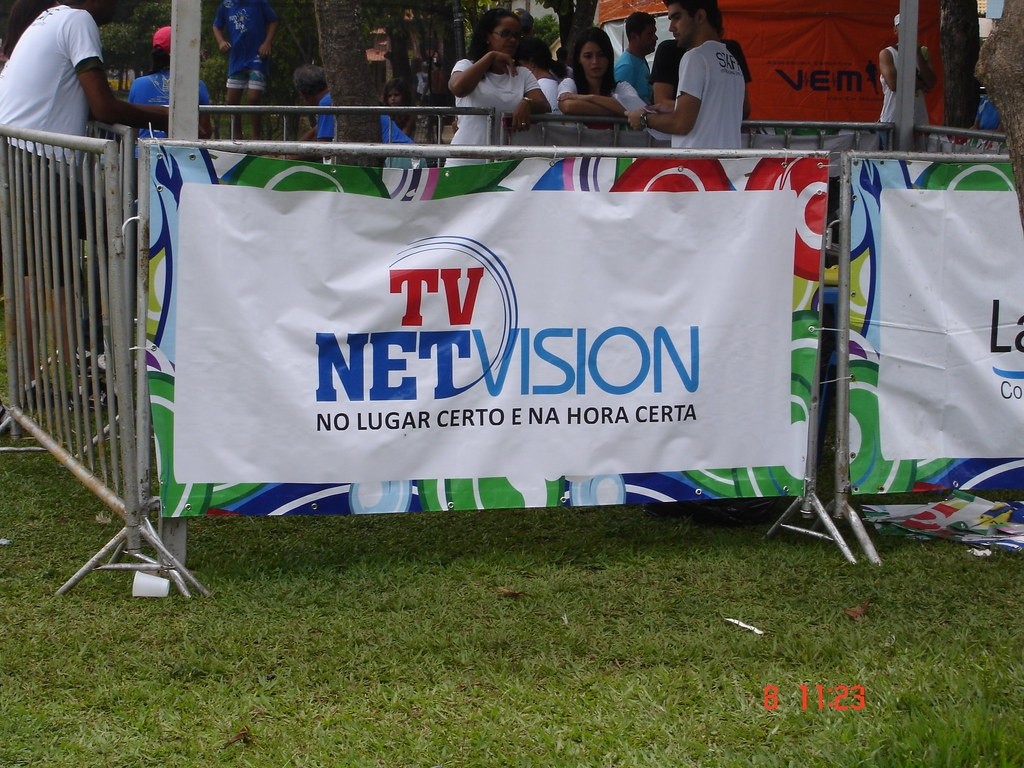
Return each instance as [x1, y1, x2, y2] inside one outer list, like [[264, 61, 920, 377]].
[[522, 122, 526, 125]]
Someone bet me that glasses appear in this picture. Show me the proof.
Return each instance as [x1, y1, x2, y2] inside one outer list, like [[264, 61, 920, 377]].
[[492, 30, 525, 41]]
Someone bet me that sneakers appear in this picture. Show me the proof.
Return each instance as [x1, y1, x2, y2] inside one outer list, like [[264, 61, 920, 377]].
[[23, 382, 72, 408], [76, 345, 117, 379], [69, 381, 120, 409]]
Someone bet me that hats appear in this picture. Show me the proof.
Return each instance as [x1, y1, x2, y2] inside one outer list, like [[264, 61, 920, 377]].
[[894, 14, 900, 26], [153, 26, 171, 53]]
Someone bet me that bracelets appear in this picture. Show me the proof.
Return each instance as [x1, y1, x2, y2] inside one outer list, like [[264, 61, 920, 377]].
[[523, 97, 533, 104]]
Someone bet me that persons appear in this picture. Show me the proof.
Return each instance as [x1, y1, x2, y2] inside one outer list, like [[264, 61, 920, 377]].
[[878, 13, 936, 127], [443, 0, 751, 171], [415, 61, 457, 132], [280, 64, 428, 170], [1, 0, 207, 412], [383, 78, 416, 138], [213, 0, 279, 142], [968, 97, 1004, 132], [128, 27, 210, 175]]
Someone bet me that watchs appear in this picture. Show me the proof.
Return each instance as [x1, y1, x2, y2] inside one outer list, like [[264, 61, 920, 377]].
[[640, 112, 649, 128]]
